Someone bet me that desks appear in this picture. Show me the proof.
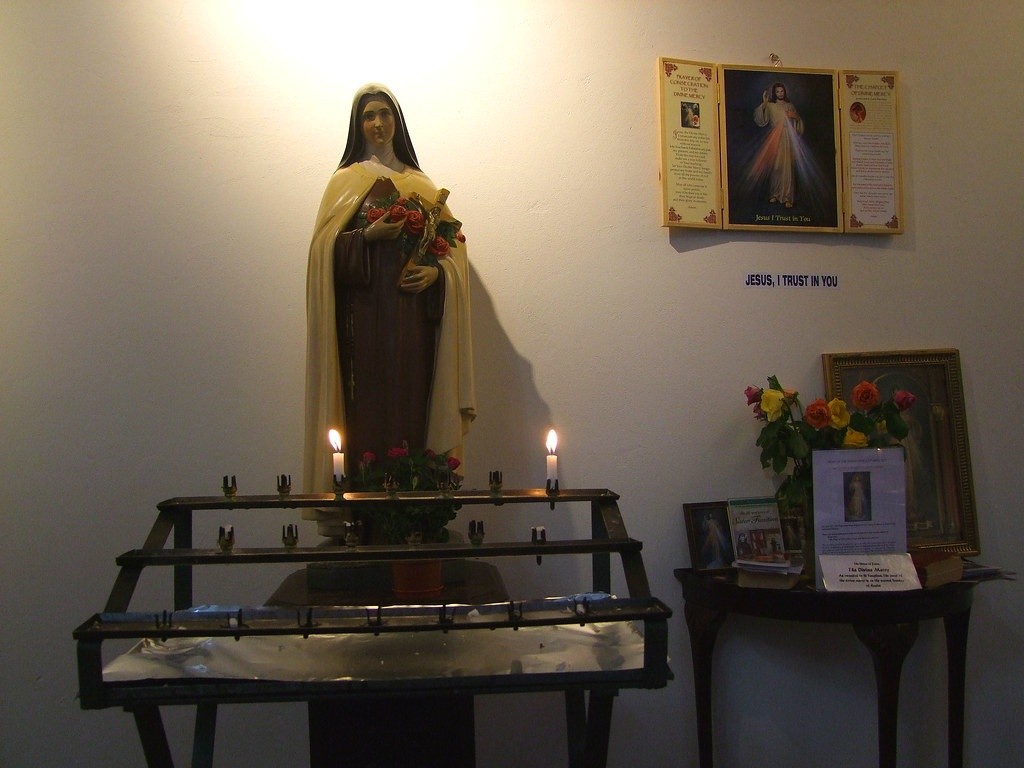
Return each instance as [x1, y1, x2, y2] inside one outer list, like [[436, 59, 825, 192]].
[[673, 567, 978, 768]]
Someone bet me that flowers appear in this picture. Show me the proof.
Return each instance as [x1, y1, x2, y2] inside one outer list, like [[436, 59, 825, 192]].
[[352, 445, 465, 545], [359, 189, 466, 267], [742, 372, 919, 518]]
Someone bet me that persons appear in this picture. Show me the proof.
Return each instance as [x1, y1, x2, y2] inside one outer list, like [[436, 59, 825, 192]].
[[296, 84, 480, 548]]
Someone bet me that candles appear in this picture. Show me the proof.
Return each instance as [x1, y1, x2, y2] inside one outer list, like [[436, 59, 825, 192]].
[[327, 428, 345, 482], [544, 428, 561, 490]]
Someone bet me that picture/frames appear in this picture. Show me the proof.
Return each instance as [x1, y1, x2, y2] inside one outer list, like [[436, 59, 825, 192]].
[[819, 348, 982, 558], [681, 500, 735, 577]]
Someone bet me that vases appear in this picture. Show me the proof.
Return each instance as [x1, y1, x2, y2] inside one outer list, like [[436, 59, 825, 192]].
[[803, 485, 818, 591], [384, 555, 449, 602]]
